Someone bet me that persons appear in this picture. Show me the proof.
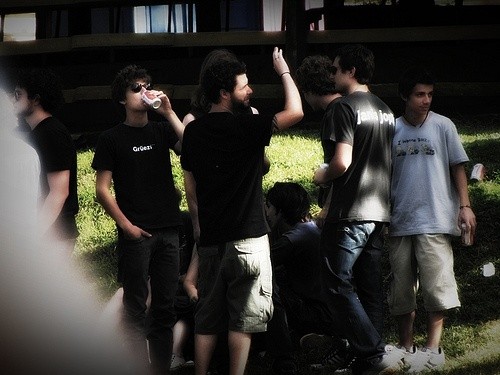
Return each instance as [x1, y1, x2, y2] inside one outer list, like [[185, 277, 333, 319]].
[[312, 44, 400, 375], [292, 55, 346, 230], [170, 185, 206, 375], [183, 49, 271, 178], [0, 91, 41, 240], [247, 181, 350, 375], [180, 46, 305, 375], [92, 65, 185, 375], [11, 69, 81, 263], [386, 72, 476, 373]]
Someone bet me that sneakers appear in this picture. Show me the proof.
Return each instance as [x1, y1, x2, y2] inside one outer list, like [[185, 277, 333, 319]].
[[383, 345, 418, 369], [408, 347, 446, 374]]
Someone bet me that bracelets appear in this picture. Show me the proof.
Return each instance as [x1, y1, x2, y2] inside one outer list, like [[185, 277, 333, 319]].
[[280, 71, 292, 78], [459, 205, 471, 209]]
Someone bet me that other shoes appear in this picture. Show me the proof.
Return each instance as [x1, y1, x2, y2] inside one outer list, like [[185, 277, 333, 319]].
[[170, 355, 185, 371], [309, 346, 355, 370], [300, 333, 329, 349], [335, 350, 382, 375]]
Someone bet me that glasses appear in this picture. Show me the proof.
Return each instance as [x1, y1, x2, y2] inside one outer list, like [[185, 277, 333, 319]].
[[328, 66, 349, 75], [14, 90, 27, 101], [127, 83, 153, 93]]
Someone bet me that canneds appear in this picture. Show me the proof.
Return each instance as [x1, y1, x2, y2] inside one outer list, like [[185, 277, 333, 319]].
[[319, 163, 331, 188], [470, 163, 485, 182], [461, 222, 473, 246], [142, 90, 162, 109]]
